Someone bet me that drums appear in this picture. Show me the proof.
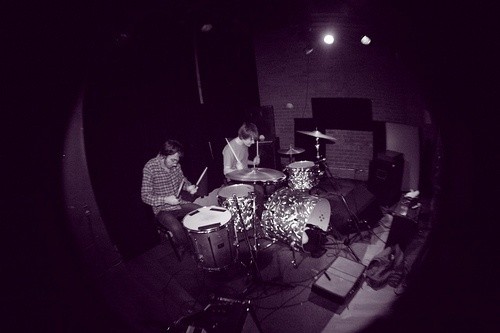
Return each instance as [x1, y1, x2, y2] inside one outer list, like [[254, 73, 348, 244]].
[[285, 160, 320, 191], [217, 185, 254, 230], [260, 187, 330, 253], [181, 205, 239, 272]]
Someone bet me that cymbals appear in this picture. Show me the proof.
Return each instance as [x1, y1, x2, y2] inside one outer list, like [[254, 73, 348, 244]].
[[278, 147, 305, 155], [297, 130, 336, 140], [226, 167, 284, 181]]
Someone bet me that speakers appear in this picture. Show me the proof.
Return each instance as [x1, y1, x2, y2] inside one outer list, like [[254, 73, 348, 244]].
[[170, 296, 262, 333], [250, 137, 280, 169], [372, 150, 403, 207]]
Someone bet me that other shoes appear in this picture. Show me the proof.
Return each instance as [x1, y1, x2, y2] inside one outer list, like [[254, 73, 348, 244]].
[[181, 255, 193, 269]]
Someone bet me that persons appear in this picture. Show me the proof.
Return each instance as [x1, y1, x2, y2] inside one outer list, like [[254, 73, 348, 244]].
[[141, 139, 202, 252], [223, 122, 276, 237]]
